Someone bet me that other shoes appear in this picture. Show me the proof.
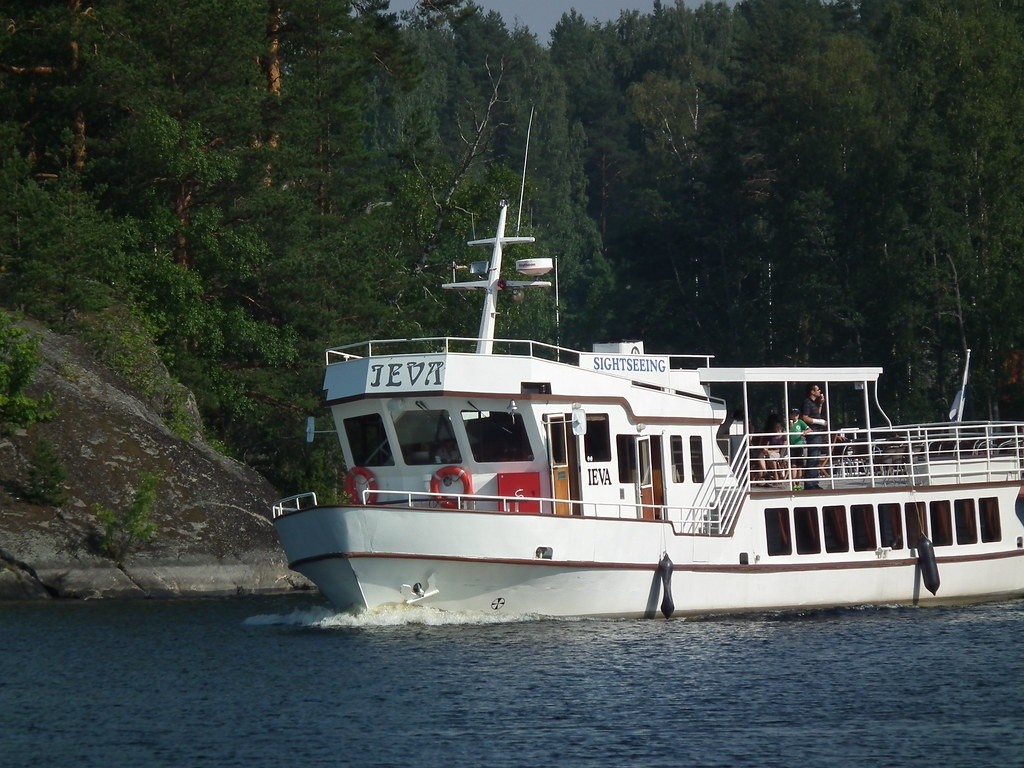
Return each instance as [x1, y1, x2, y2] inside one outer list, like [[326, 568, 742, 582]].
[[793, 486, 801, 491], [804, 485, 823, 489]]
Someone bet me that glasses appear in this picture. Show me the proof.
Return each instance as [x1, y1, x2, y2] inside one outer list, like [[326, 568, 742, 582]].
[[815, 389, 820, 391]]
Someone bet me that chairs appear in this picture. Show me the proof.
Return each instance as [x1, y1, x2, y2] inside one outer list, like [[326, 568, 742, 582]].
[[750, 439, 925, 488]]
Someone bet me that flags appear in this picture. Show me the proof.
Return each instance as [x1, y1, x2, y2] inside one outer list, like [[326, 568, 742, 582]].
[[949, 359, 968, 421]]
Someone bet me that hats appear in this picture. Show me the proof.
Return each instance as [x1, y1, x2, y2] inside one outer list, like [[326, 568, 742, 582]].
[[790, 408, 800, 414]]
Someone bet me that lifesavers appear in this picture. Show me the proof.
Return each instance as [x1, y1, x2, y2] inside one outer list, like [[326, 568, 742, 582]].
[[344, 465, 378, 507], [429, 464, 473, 511]]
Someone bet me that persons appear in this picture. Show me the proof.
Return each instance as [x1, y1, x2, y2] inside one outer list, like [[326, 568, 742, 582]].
[[803, 383, 830, 491], [759, 421, 787, 485], [788, 407, 812, 492], [748, 411, 756, 487]]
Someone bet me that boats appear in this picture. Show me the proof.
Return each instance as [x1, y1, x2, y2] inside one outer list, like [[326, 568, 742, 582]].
[[272, 105, 1024, 626]]
